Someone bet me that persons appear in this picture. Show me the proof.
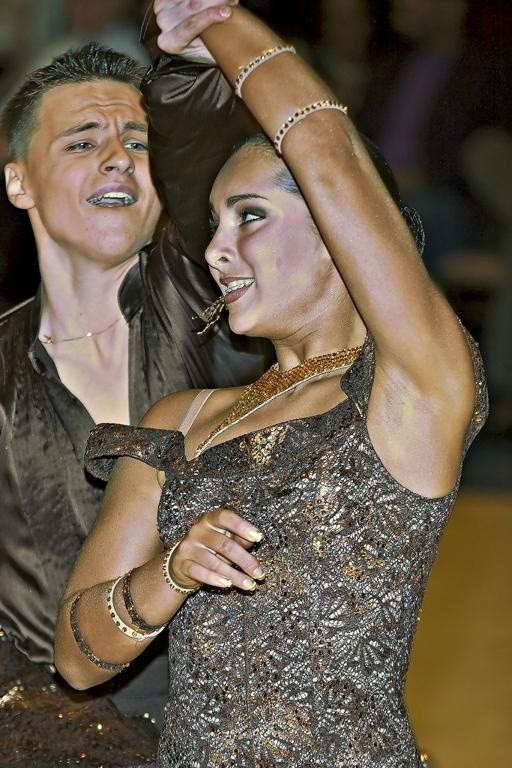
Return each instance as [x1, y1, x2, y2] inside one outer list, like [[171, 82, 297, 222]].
[[321, 1, 512, 487], [0, 0, 239, 768], [55, 0, 488, 768]]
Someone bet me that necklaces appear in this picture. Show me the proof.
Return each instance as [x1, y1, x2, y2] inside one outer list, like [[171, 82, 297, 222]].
[[40, 318, 122, 344], [196, 345, 362, 458]]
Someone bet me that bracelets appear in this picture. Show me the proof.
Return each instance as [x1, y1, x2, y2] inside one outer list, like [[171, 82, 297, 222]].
[[120, 565, 165, 634], [272, 100, 350, 155], [67, 592, 132, 673], [164, 537, 200, 595], [104, 574, 166, 642], [234, 43, 297, 99]]
